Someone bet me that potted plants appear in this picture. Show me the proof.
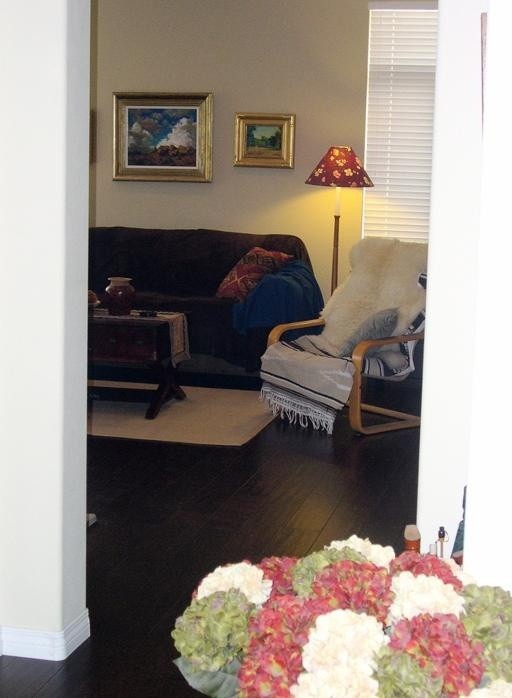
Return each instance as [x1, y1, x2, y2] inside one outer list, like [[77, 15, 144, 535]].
[[110, 90, 217, 186], [232, 111, 296, 171]]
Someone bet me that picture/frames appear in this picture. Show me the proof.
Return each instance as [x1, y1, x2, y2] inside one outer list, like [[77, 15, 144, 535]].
[[86, 305, 193, 420]]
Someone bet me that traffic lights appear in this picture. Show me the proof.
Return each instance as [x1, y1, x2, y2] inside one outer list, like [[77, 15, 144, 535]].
[[343, 308, 396, 361], [214, 246, 289, 305]]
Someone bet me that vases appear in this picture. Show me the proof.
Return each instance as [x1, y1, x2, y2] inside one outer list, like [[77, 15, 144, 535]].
[[167, 529, 511, 697]]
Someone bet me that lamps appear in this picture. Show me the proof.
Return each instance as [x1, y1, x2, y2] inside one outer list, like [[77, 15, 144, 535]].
[[258, 229, 428, 437]]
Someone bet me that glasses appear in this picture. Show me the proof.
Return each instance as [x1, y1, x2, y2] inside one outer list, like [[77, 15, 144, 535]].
[[301, 145, 376, 297]]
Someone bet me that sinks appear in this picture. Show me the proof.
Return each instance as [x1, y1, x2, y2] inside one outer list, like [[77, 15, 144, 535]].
[[87, 219, 324, 392]]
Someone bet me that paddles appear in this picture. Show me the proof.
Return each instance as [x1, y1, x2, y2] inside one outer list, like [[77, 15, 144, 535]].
[[87, 370, 287, 449]]
